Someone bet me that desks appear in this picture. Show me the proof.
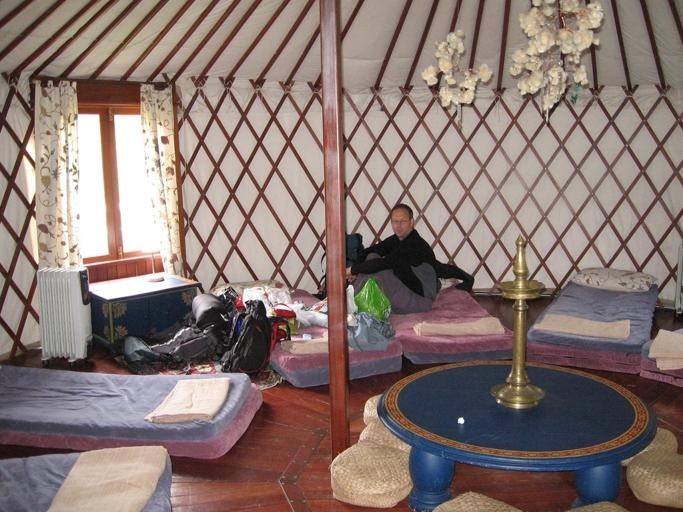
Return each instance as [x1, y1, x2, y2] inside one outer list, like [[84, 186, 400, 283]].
[[375, 361, 659, 512]]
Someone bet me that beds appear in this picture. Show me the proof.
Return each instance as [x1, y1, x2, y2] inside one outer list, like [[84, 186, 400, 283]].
[[1, 364, 262, 512]]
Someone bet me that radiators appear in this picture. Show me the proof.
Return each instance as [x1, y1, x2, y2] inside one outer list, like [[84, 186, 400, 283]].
[[38, 265, 94, 368]]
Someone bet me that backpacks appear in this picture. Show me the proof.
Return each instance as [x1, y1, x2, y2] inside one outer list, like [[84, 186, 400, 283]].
[[317, 234, 363, 299], [222, 299, 270, 373]]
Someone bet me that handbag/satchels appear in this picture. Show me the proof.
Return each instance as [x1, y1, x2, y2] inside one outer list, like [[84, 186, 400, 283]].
[[347, 311, 393, 351], [354, 278, 391, 318]]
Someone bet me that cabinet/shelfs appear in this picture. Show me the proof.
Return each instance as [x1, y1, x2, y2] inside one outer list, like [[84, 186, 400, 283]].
[[89, 271, 202, 353]]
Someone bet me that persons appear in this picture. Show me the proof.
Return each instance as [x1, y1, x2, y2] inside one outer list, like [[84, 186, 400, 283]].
[[344, 204, 438, 314]]
